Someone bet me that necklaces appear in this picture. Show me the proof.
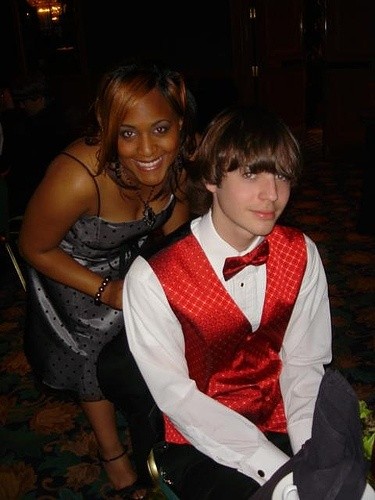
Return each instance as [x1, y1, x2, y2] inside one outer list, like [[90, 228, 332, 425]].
[[120, 167, 155, 227]]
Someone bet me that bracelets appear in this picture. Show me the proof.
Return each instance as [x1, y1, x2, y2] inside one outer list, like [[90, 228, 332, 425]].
[[94, 276, 112, 307]]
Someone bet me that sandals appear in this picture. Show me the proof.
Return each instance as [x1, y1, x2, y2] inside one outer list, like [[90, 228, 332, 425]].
[[98, 445, 150, 500]]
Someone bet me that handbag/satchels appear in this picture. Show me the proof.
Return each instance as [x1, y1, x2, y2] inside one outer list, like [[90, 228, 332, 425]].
[[250, 369, 366, 499]]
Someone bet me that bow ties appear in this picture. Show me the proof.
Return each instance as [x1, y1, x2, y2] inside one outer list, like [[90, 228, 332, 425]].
[[222, 240, 270, 282]]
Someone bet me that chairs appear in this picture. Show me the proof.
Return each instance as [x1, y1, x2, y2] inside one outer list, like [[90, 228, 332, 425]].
[[96, 326, 259, 499], [0, 182, 28, 293]]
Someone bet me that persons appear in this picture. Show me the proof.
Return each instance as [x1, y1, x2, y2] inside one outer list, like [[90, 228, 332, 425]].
[[122, 107, 375, 500], [17, 59, 202, 500], [0, 72, 67, 218]]
